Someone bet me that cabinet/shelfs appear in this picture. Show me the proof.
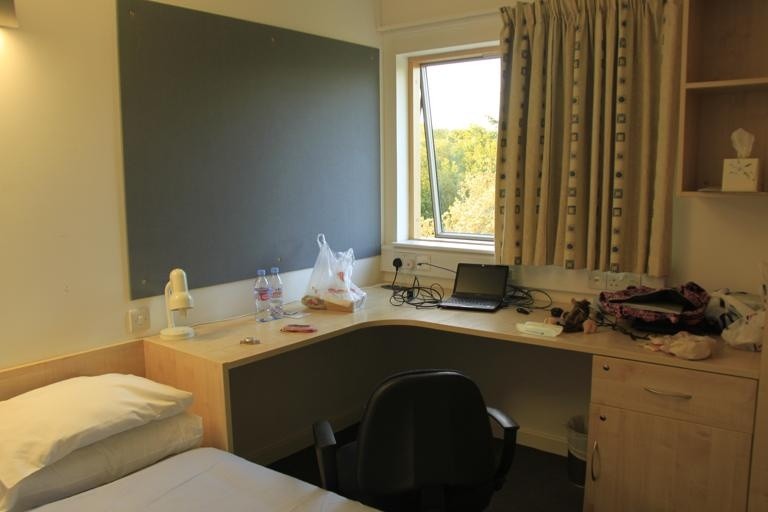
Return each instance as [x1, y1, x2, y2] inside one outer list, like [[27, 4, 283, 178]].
[[673, 0, 768, 196]]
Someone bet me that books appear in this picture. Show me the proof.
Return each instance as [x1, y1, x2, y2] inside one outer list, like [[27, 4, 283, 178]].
[[623, 301, 682, 315]]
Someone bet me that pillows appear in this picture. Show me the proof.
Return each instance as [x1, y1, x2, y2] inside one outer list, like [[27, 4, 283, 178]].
[[0, 411, 204, 511], [0, 372, 195, 491]]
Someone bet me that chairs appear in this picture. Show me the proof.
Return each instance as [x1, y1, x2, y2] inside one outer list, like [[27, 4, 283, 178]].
[[311, 369, 519, 511]]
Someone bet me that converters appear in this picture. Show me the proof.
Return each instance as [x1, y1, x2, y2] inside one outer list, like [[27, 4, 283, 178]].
[[407, 288, 414, 298]]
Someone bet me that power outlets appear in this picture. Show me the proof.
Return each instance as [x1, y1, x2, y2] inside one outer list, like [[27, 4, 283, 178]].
[[391, 252, 417, 271], [606, 271, 627, 291]]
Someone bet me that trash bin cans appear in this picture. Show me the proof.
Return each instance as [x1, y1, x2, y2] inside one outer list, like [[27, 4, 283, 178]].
[[567, 414, 589, 489]]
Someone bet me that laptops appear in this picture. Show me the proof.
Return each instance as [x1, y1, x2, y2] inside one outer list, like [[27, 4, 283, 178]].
[[439, 263, 509, 312]]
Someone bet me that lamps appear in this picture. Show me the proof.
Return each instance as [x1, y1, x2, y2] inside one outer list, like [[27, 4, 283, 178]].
[[160, 269, 197, 339]]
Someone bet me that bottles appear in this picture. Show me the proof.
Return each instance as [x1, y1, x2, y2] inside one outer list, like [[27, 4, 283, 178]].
[[253, 270, 273, 325], [268, 266, 285, 317]]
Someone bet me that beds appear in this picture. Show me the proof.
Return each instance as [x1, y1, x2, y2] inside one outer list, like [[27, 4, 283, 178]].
[[0, 339, 380, 511]]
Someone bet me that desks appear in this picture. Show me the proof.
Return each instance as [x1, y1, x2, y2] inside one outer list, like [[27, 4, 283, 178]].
[[141, 281, 767, 509]]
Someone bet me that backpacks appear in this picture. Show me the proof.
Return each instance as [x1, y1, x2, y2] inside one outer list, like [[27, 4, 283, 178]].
[[596, 281, 712, 331]]
